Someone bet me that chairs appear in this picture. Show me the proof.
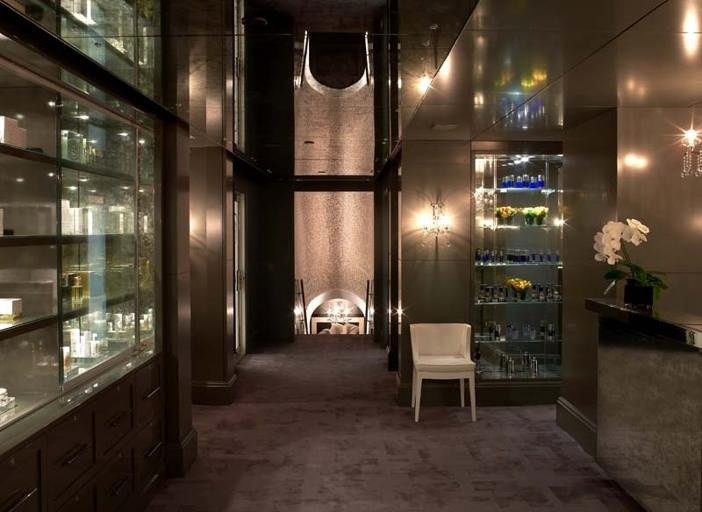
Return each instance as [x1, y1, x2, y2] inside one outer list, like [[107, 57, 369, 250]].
[[407, 323, 478, 422]]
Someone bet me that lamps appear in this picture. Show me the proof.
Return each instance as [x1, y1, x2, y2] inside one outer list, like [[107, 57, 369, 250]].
[[675, 123, 702, 178]]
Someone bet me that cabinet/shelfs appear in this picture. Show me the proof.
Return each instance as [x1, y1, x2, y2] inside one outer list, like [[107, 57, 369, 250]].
[[466, 140, 565, 407], [1, 2, 169, 510]]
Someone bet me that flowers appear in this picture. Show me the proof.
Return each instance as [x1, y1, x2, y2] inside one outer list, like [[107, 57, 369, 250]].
[[593, 219, 667, 301]]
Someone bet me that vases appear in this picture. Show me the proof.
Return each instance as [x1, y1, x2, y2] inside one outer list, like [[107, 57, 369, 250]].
[[622, 283, 653, 305]]
